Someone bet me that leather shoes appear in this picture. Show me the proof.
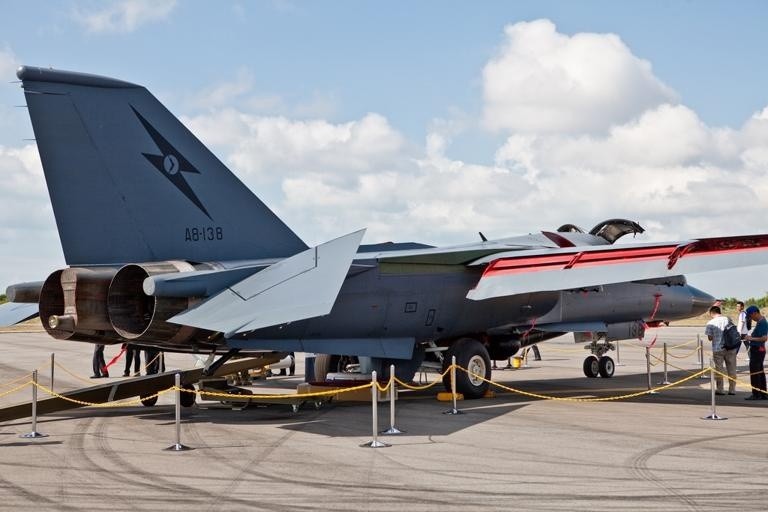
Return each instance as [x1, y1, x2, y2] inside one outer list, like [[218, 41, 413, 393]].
[[745, 394, 759, 400]]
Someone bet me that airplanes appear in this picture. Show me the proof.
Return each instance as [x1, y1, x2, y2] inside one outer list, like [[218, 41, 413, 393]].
[[0, 65, 767, 400]]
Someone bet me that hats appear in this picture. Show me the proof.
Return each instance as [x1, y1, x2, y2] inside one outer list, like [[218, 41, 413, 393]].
[[746, 306, 759, 313]]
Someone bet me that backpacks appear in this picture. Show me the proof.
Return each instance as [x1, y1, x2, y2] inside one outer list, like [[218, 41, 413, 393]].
[[724, 316, 742, 350]]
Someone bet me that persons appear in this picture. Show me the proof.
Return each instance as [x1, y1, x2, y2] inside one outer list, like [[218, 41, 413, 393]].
[[706, 305, 737, 395], [122, 349, 140, 377], [89, 343, 109, 378], [744, 305, 767, 401], [519, 344, 541, 361], [278, 351, 295, 376], [735, 302, 750, 355]]
